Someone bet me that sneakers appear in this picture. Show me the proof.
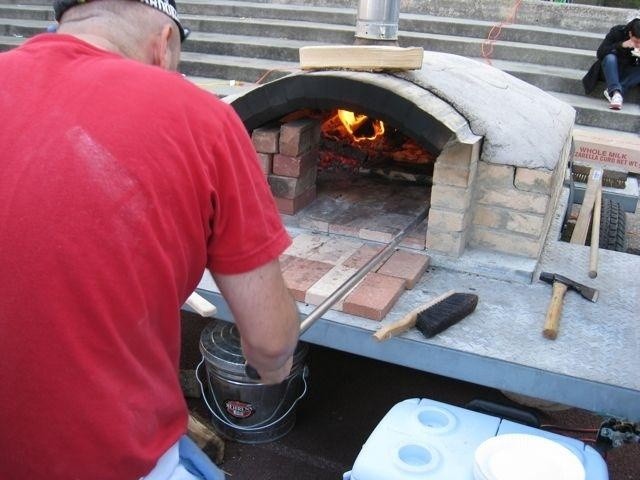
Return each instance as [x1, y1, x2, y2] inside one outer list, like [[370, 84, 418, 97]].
[[603, 88, 612, 102], [608, 92, 623, 110]]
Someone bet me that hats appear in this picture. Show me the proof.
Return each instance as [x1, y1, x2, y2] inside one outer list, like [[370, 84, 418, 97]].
[[52, 1, 190, 42]]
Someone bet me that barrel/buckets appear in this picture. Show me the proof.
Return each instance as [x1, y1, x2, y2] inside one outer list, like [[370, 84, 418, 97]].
[[194, 318, 310, 445]]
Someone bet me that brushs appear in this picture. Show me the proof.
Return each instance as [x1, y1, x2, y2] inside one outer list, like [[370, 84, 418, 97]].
[[373, 292, 479, 344]]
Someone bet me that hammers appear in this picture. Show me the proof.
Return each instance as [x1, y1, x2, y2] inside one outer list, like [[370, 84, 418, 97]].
[[540, 272, 598, 341]]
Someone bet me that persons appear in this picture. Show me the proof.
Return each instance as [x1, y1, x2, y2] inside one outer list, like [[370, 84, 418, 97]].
[[0, 0, 302, 480], [582, 17, 640, 110]]
